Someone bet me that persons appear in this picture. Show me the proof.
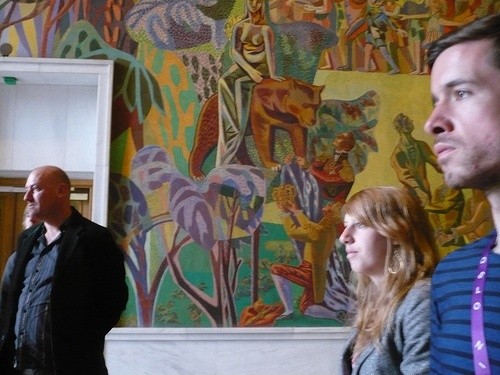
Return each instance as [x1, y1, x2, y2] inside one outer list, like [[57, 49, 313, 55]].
[[1, 203, 42, 345], [423, 7, 500, 375], [4, 164, 129, 375], [335, 184, 442, 375]]
